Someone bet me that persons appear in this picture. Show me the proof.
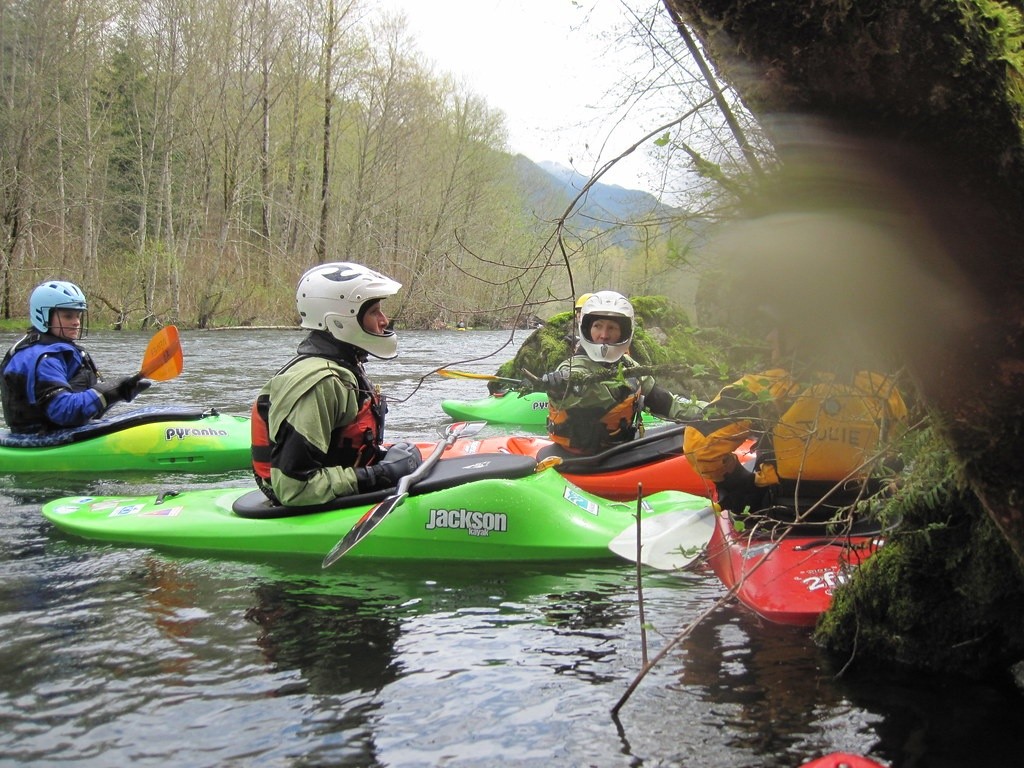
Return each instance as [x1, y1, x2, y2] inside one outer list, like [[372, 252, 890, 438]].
[[0, 281, 152, 434], [682, 311, 908, 537], [459, 321, 466, 328], [251, 262, 422, 507], [541, 291, 728, 460]]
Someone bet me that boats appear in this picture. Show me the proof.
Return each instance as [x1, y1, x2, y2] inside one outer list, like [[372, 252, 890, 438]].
[[42, 457, 720, 567], [701, 433, 890, 629], [382, 424, 719, 509], [796, 752, 893, 768], [0, 404, 255, 479], [437, 391, 664, 432]]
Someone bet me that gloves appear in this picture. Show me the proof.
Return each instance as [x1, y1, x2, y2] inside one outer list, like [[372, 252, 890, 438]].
[[355, 441, 423, 495], [712, 461, 768, 513], [91, 374, 152, 421], [542, 371, 573, 399]]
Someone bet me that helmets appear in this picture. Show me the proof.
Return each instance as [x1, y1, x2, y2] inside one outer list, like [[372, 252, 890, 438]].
[[574, 290, 635, 364], [30, 279, 88, 341], [296, 261, 402, 361]]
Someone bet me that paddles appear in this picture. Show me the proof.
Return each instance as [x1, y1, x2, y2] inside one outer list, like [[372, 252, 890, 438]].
[[321, 420, 489, 569], [132, 325, 183, 381], [436, 368, 520, 385], [608, 494, 727, 571], [534, 425, 688, 472]]
